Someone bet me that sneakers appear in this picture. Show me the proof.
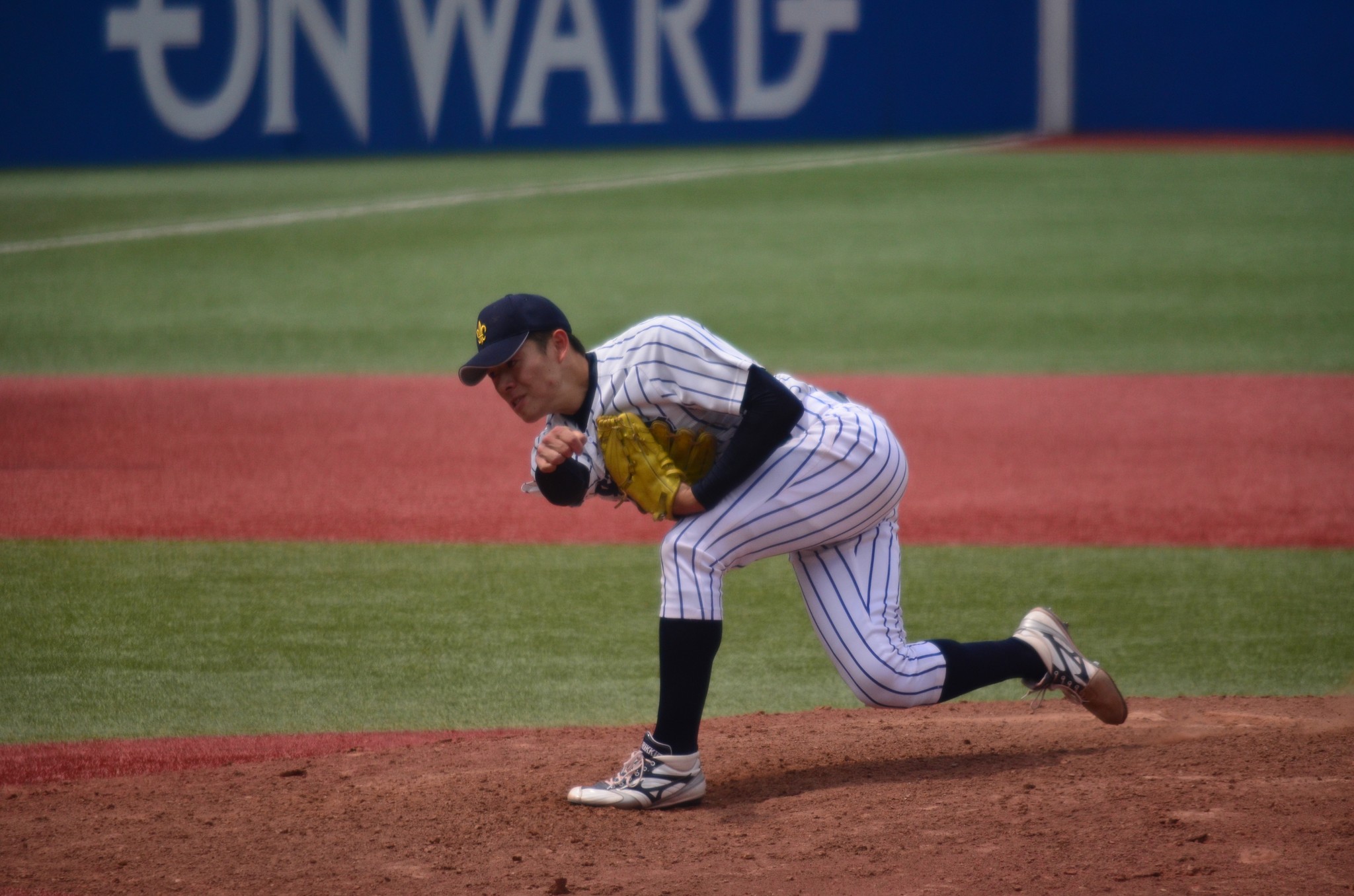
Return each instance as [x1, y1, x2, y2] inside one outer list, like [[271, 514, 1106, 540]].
[[566, 730, 706, 808], [1012, 606, 1127, 725]]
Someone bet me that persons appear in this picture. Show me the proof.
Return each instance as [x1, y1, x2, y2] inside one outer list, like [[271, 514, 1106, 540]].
[[459, 295, 1127, 809]]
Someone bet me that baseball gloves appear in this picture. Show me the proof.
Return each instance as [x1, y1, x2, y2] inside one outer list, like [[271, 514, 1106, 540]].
[[596, 412, 716, 520]]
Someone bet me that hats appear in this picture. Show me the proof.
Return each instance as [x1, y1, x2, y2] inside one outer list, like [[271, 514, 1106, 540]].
[[458, 293, 572, 385]]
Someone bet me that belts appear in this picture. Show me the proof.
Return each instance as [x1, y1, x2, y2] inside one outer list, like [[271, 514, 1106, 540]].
[[826, 389, 848, 404]]
